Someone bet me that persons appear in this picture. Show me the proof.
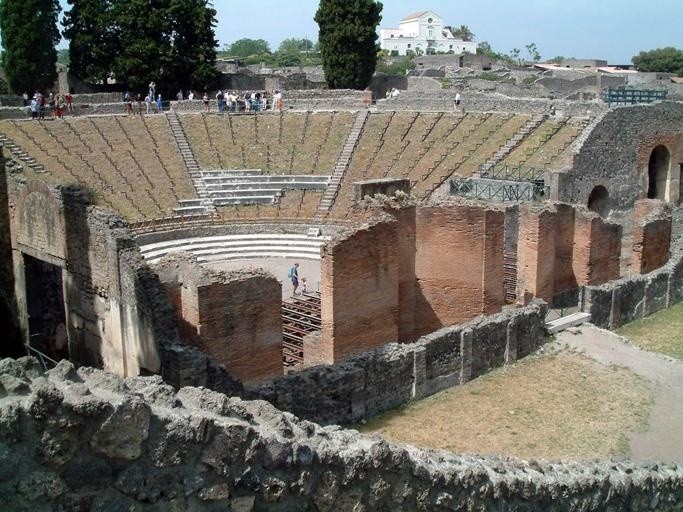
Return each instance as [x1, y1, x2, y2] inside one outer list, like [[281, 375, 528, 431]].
[[453, 90, 461, 113], [385, 88, 391, 101], [22, 77, 282, 121], [389, 88, 400, 99], [288, 263, 299, 295], [300, 278, 306, 295]]
